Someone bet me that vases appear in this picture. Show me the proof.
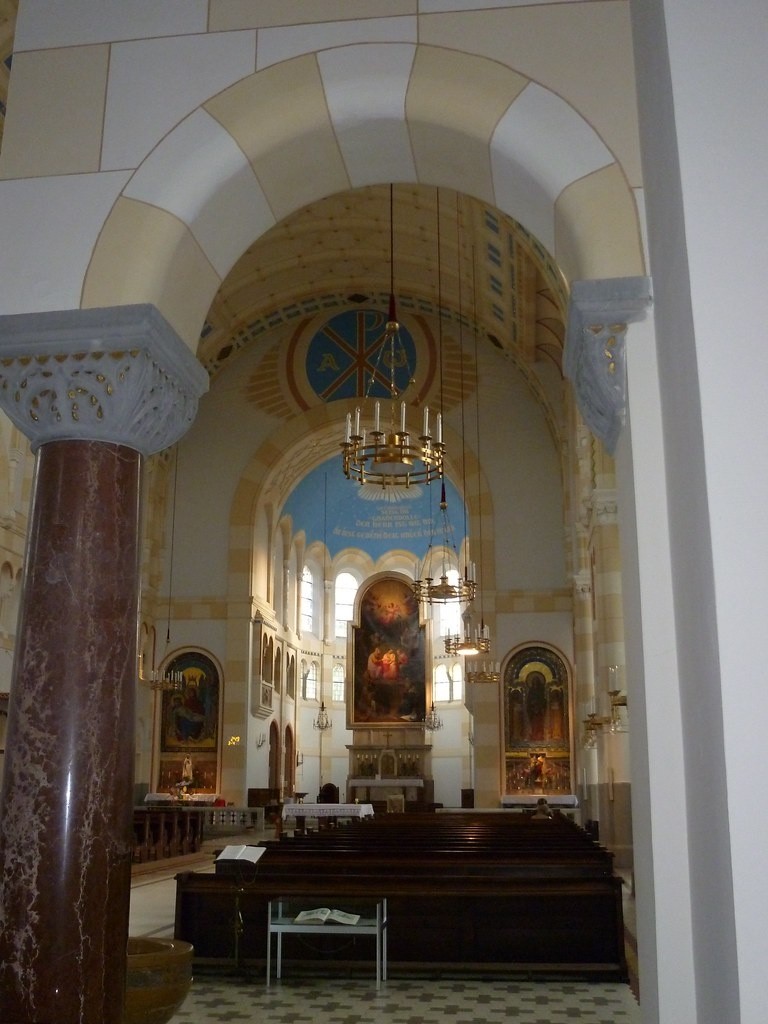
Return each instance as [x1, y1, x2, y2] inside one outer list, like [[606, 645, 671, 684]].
[[125, 936, 195, 1024]]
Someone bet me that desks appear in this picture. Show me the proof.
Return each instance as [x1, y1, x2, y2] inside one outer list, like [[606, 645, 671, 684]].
[[282, 804, 375, 832]]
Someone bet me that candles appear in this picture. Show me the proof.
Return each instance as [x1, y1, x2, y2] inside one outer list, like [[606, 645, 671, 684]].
[[584, 696, 598, 713], [357, 752, 419, 764], [607, 664, 622, 691]]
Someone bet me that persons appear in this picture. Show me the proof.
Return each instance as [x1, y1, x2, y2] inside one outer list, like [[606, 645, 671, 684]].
[[530, 798, 553, 819]]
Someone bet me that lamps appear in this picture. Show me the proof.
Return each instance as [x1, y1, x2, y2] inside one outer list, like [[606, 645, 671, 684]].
[[444, 359, 490, 658], [339, 185, 447, 490], [312, 700, 333, 731], [146, 442, 184, 693], [422, 701, 443, 734], [411, 312, 479, 608], [465, 366, 500, 683]]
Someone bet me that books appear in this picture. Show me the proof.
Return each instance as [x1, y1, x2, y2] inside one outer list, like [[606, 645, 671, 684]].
[[217, 845, 266, 864], [292, 908, 360, 926]]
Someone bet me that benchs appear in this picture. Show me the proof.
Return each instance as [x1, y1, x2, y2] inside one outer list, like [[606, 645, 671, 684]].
[[172, 809, 631, 983], [134, 805, 204, 864]]
